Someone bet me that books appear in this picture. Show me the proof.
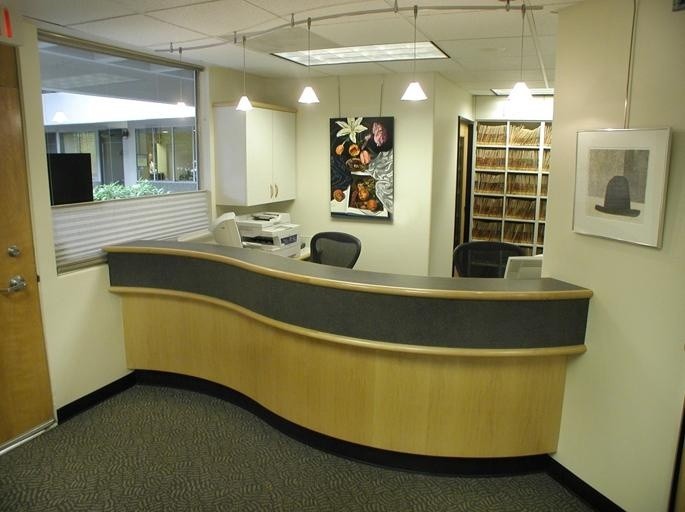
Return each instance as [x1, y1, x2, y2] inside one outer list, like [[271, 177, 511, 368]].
[[472, 124, 553, 243]]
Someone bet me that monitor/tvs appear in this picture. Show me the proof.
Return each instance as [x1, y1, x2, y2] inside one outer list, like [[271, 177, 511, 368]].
[[504, 256, 543, 280], [177, 212, 243, 248]]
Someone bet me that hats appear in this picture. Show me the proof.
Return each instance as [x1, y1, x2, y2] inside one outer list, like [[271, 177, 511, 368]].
[[596, 176, 640, 217]]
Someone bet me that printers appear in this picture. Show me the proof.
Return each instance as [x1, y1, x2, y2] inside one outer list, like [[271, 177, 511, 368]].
[[236, 212, 301, 257]]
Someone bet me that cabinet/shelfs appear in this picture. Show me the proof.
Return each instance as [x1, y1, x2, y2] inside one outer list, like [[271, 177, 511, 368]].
[[211, 100, 297, 208], [471, 118, 553, 258]]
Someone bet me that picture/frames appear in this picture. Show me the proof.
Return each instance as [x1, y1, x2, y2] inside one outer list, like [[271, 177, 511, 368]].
[[569, 127, 674, 249]]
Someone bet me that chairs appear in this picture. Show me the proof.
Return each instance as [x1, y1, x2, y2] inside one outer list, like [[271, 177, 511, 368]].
[[310, 232, 361, 269], [453, 242, 527, 277]]
